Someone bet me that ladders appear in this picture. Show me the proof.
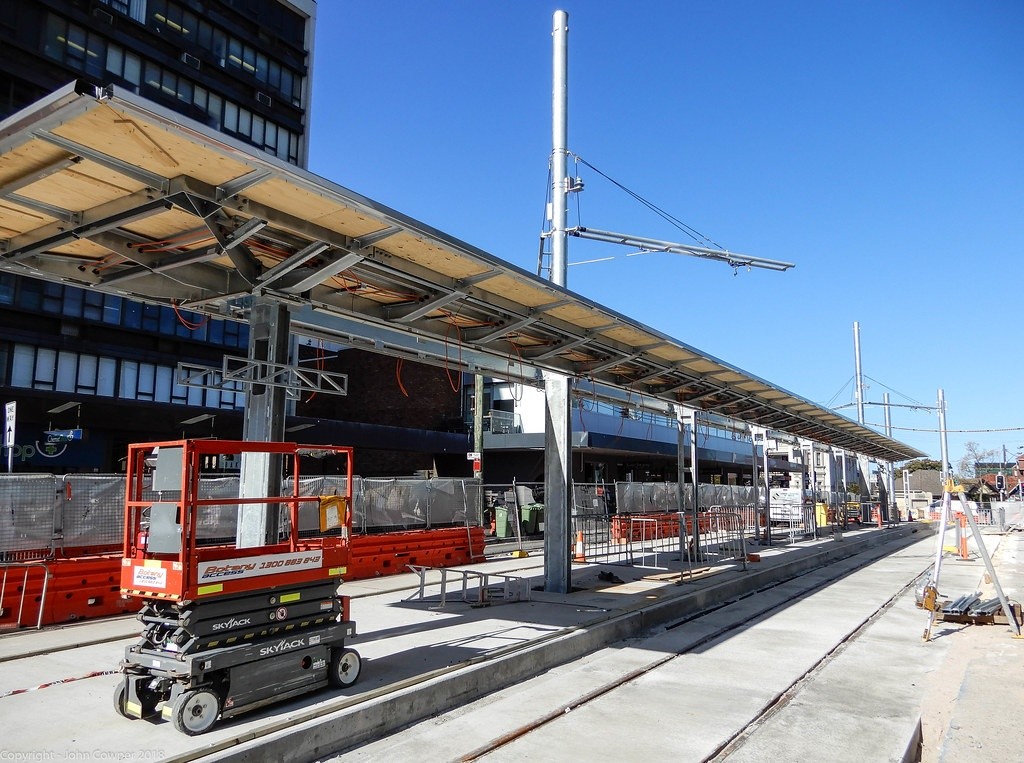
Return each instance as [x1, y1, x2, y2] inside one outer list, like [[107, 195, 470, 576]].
[[401, 564, 531, 610]]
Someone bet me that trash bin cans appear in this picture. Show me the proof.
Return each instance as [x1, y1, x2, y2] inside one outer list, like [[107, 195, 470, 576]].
[[495, 507, 512, 538], [816, 503, 828, 525], [521, 505, 539, 536], [531, 503, 544, 535], [889, 502, 898, 523]]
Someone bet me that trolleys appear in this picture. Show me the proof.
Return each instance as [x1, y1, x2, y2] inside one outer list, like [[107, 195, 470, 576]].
[[112, 441, 362, 736]]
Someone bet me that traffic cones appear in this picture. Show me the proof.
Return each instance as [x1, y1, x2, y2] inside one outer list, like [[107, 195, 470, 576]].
[[573, 530, 590, 564]]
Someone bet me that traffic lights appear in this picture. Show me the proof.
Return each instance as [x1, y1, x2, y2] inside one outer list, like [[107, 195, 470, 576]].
[[996, 476, 1005, 489]]
